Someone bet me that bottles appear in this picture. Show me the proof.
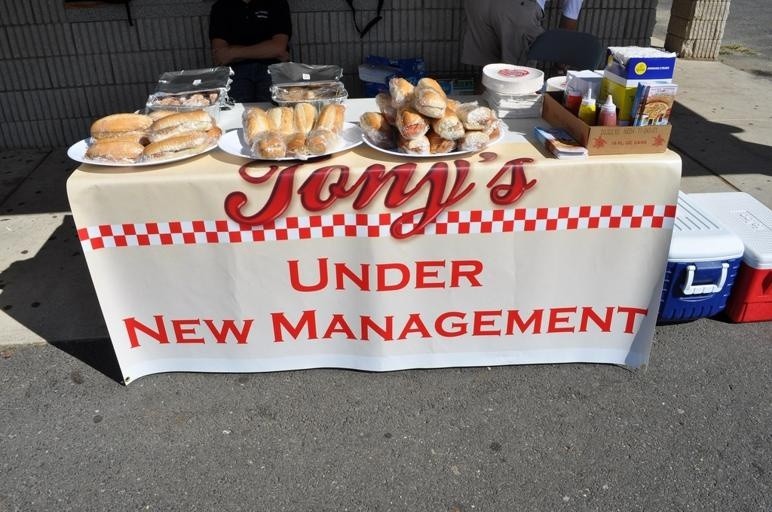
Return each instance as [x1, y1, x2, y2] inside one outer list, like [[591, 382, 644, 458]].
[[600, 94, 618, 126], [578, 87, 597, 124]]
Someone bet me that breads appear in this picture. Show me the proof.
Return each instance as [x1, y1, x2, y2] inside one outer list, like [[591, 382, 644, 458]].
[[242, 86, 344, 158], [360, 77, 500, 155], [84, 93, 221, 163]]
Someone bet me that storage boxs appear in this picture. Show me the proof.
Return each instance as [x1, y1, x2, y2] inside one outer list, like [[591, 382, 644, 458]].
[[543, 92, 673, 157], [658, 190, 772, 325]]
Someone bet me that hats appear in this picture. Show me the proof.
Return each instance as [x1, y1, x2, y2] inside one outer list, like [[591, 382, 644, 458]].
[[562, 0, 584, 21], [538, 0, 547, 8]]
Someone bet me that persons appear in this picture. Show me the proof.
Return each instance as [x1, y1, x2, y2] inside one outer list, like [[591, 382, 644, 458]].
[[460, 0, 583, 95], [208, 0, 291, 104]]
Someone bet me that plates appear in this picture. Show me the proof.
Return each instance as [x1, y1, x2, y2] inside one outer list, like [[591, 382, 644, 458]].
[[66, 130, 224, 169], [362, 120, 507, 157], [219, 121, 363, 161], [546, 76, 568, 92], [481, 63, 545, 93]]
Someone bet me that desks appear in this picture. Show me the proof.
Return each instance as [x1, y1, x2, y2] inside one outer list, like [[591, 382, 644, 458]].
[[66, 97, 682, 387]]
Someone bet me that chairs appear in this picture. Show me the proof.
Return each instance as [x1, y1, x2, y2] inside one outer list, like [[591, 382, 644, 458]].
[[524, 27, 606, 76]]
